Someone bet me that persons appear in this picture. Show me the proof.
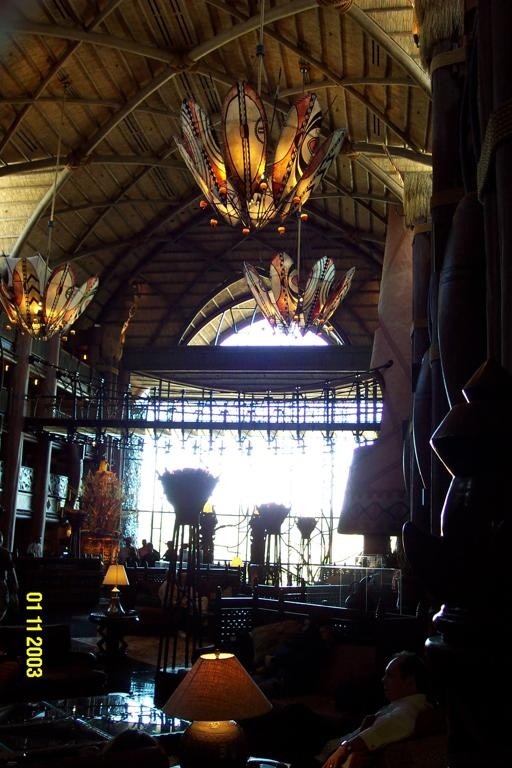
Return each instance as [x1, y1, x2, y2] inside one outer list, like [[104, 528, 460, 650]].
[[26, 536, 42, 557], [117, 538, 177, 566], [159, 568, 199, 640], [100, 729, 158, 753], [0, 531, 19, 620], [321, 650, 440, 768]]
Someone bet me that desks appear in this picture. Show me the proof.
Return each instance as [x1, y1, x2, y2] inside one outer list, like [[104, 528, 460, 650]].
[[0, 695, 191, 768]]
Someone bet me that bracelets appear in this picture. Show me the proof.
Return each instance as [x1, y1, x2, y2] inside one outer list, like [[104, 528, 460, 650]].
[[341, 740, 352, 754]]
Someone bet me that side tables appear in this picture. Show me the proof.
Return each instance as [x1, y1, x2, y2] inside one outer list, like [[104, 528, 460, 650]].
[[89, 614, 139, 670]]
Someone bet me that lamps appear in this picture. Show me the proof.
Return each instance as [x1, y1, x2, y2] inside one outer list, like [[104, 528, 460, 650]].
[[162, 649, 274, 766], [173, 0, 347, 236], [0, 72, 101, 342], [244, 207, 359, 337], [103, 563, 132, 616]]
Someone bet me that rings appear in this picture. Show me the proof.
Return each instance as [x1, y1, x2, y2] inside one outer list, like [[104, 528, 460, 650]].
[[329, 764, 333, 768]]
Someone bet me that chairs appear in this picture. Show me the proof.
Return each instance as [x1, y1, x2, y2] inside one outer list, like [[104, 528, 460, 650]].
[[348, 709, 450, 768]]
